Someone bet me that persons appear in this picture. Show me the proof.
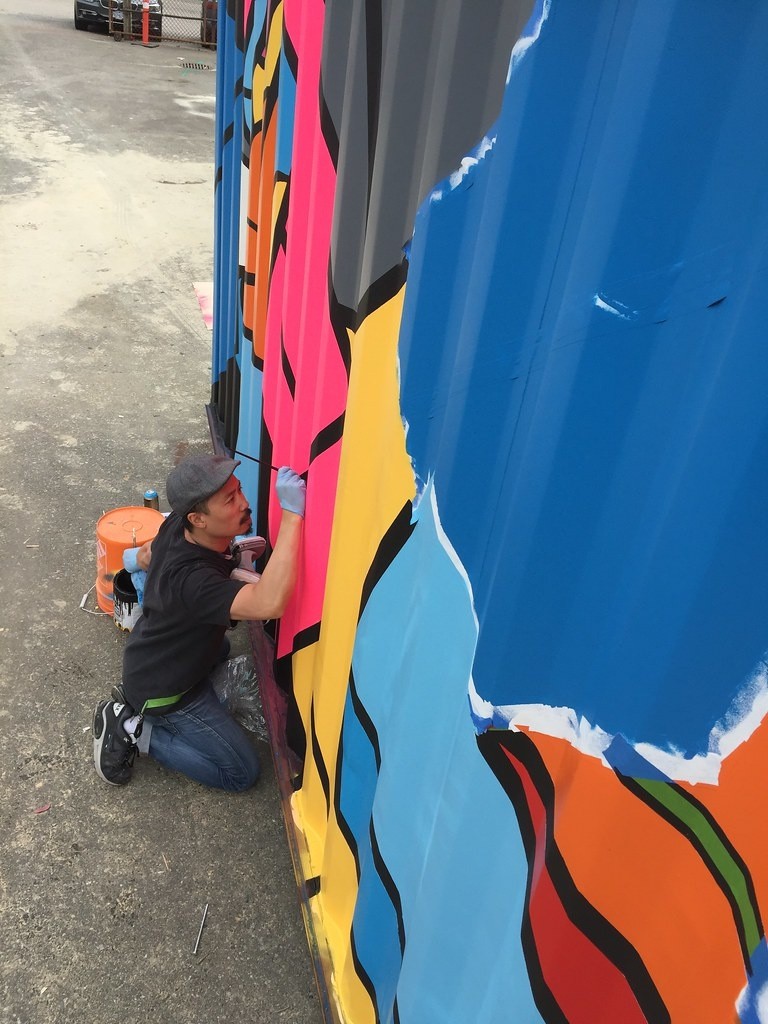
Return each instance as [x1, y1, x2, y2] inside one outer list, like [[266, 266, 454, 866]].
[[91, 453, 307, 793]]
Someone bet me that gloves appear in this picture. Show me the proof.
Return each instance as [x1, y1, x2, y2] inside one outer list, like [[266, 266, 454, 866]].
[[122, 547, 142, 574], [275, 465, 307, 519]]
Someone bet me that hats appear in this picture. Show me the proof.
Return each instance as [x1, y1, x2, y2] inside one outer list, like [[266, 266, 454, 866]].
[[166, 454, 242, 519]]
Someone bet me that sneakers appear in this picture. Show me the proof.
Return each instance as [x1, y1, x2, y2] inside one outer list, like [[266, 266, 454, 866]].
[[92, 700, 140, 787], [110, 683, 127, 704]]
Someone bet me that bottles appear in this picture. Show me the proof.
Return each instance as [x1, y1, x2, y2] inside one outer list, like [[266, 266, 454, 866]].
[[144, 490, 160, 512]]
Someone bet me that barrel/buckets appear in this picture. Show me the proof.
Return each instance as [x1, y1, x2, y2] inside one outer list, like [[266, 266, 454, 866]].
[[112, 568, 143, 635], [80, 505, 168, 617]]
